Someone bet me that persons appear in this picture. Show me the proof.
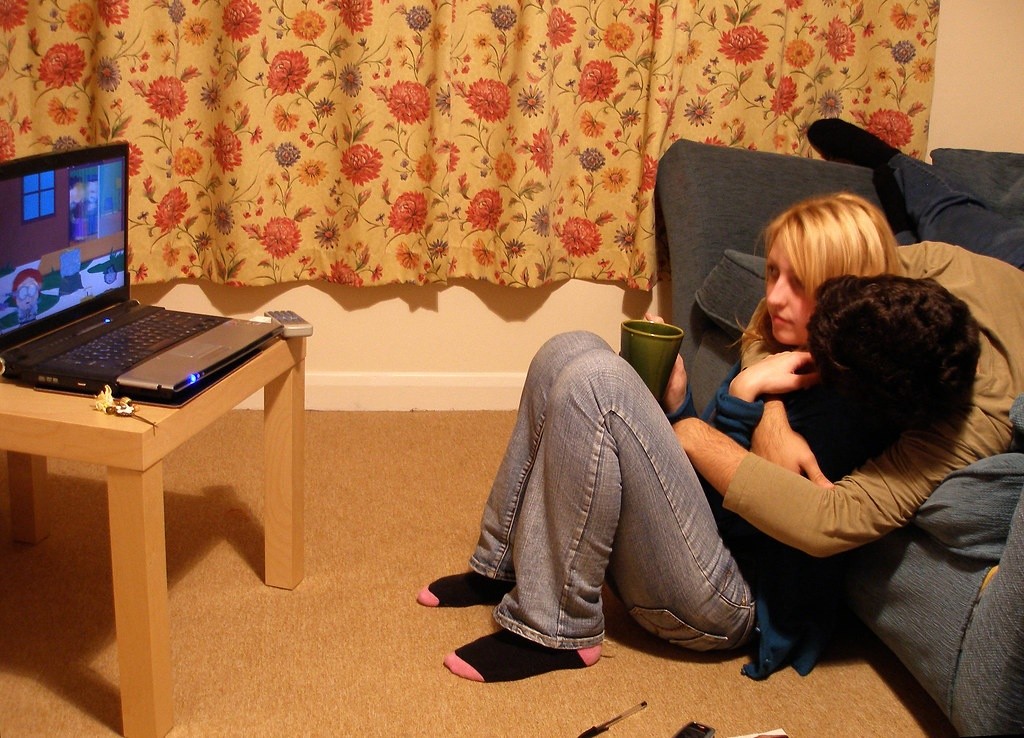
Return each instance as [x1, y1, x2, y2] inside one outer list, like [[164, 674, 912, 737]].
[[672, 116, 1023, 554], [416, 190, 899, 684]]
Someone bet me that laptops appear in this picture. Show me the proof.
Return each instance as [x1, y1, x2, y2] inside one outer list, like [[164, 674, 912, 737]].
[[0, 139, 284, 393]]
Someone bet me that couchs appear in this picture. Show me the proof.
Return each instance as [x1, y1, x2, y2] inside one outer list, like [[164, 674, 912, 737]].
[[655, 119, 1024, 738]]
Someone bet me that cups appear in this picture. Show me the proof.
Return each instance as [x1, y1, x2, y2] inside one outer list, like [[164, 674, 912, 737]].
[[620, 320, 684, 403]]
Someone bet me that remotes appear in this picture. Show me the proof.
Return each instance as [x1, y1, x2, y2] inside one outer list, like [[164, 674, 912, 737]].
[[264, 311, 314, 338]]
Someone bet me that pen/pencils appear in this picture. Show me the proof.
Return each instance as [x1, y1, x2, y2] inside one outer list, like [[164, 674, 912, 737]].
[[577, 701, 648, 738]]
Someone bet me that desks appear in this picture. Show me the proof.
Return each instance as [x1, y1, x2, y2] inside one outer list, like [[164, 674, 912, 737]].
[[0, 337, 307, 738]]
